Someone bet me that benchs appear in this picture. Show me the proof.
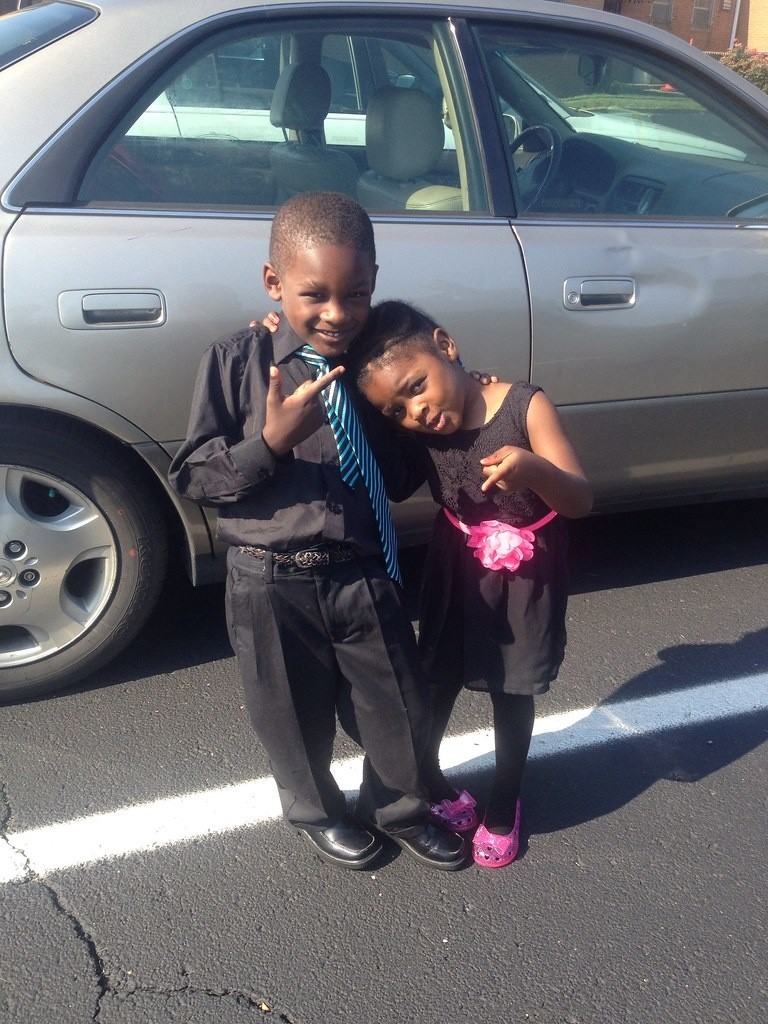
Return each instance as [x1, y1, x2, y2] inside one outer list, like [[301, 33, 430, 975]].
[[96, 145, 170, 200]]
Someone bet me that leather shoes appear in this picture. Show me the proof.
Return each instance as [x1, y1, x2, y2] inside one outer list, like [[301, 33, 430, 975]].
[[287, 812, 382, 873], [354, 797, 468, 871]]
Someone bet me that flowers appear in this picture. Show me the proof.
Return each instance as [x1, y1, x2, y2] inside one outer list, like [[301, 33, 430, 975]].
[[467, 519, 537, 570]]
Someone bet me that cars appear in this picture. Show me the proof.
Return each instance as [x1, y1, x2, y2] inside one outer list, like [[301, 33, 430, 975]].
[[0, 0, 768, 706]]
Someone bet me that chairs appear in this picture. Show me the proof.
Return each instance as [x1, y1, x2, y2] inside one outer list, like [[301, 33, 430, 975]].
[[356, 86, 465, 212], [270, 63, 359, 208]]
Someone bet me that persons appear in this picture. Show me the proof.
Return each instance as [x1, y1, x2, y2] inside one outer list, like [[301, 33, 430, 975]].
[[168, 192, 499, 870], [250, 300, 593, 870]]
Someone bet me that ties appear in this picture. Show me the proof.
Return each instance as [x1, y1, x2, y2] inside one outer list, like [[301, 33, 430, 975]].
[[294, 342, 405, 589]]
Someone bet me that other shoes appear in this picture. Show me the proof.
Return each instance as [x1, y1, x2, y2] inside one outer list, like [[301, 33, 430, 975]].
[[474, 791, 521, 868], [423, 776, 477, 830]]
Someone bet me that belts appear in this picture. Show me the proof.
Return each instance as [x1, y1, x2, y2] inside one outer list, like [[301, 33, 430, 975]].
[[445, 509, 560, 572], [233, 542, 385, 570]]
